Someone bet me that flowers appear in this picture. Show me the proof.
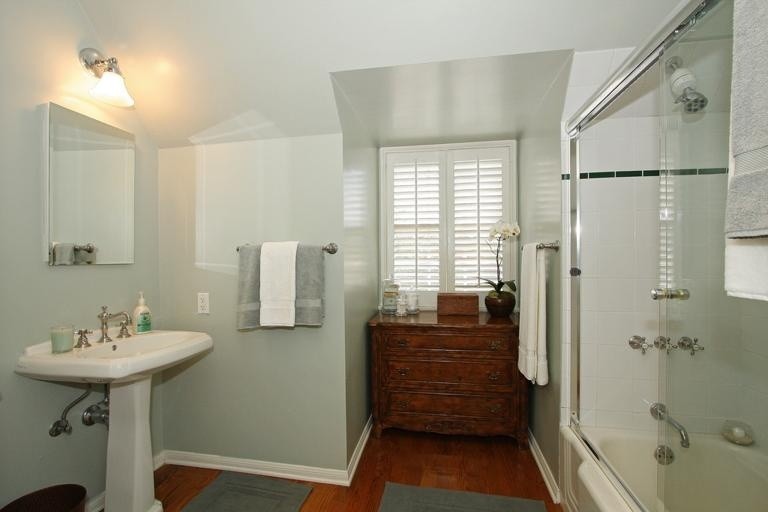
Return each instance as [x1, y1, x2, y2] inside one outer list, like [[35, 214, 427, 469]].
[[473, 219, 524, 297]]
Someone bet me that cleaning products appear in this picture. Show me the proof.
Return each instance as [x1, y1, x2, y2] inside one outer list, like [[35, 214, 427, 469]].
[[132, 291, 152, 333]]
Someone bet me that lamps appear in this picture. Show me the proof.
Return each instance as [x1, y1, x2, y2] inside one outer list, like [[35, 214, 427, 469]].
[[86, 71, 136, 109]]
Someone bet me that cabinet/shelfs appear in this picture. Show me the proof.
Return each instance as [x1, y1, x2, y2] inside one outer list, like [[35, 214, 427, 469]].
[[369, 310, 529, 448]]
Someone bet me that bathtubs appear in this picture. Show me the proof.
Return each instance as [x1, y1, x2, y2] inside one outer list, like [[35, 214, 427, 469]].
[[578, 427, 767, 512]]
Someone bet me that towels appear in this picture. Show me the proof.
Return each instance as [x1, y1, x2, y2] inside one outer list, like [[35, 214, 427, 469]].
[[258, 239, 297, 330], [234, 240, 326, 334], [518, 243, 549, 387]]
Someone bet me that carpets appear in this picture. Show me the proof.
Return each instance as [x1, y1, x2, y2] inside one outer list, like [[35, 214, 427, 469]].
[[175, 469, 312, 512], [374, 481, 549, 512]]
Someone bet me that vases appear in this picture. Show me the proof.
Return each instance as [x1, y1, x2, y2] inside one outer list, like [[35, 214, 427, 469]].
[[482, 291, 517, 320]]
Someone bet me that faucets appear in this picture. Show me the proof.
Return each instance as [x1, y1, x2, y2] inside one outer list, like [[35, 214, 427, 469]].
[[95, 303, 133, 346], [651, 402, 692, 449]]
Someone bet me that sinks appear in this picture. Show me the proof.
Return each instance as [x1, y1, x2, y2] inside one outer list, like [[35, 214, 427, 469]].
[[11, 324, 214, 384]]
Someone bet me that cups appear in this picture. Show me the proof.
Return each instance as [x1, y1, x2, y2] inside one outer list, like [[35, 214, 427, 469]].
[[382, 281, 399, 316], [405, 294, 418, 314], [51, 326, 74, 353], [397, 295, 406, 316]]
